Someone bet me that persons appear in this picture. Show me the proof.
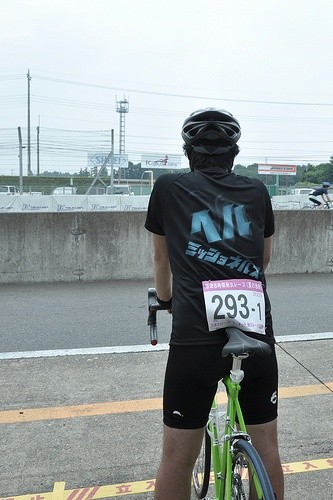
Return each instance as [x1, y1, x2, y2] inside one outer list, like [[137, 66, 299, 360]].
[[145, 108, 284, 500], [308, 182, 333, 210]]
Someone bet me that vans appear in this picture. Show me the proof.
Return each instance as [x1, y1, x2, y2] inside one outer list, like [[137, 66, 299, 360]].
[[0, 186, 20, 195], [292, 188, 315, 194]]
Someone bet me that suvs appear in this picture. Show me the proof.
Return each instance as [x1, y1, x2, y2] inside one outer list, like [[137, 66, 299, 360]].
[[104, 185, 134, 196]]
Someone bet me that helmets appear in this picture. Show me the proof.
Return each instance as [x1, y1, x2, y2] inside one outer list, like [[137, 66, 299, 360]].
[[323, 182, 330, 187], [181, 106, 241, 156]]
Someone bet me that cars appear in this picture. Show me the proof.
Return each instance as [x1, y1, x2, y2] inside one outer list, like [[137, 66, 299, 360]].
[[52, 186, 77, 195]]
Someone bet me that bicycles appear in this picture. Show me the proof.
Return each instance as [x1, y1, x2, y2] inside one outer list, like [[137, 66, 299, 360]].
[[302, 200, 333, 210], [147, 287, 277, 500]]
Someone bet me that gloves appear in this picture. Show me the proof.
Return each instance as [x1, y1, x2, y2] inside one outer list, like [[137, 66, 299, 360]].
[[157, 297, 172, 309]]
